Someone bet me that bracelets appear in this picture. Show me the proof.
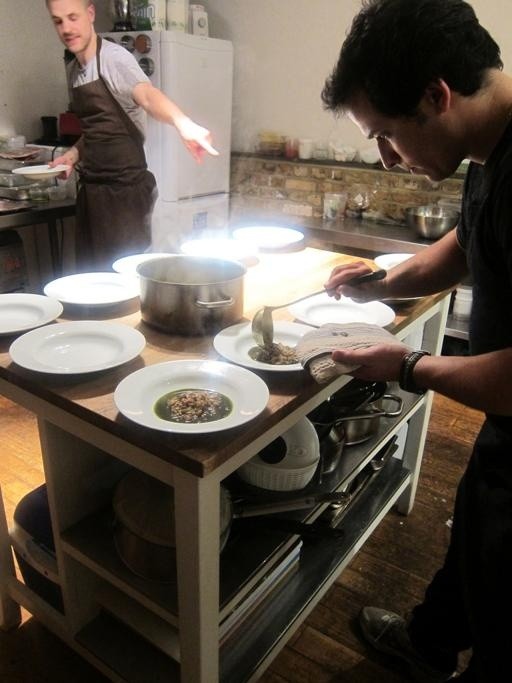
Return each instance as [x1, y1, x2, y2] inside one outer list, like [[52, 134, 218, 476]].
[[398, 349, 432, 394]]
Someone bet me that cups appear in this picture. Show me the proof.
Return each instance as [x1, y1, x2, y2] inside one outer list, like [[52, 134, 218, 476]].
[[323, 192, 347, 221], [300, 140, 313, 160], [284, 139, 298, 159]]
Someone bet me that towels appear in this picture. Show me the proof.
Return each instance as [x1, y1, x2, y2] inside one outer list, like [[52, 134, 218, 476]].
[[296, 322, 401, 385]]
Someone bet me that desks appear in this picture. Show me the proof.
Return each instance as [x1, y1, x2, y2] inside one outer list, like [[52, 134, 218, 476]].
[[0, 197, 78, 293], [0, 244, 462, 682], [294, 216, 472, 341]]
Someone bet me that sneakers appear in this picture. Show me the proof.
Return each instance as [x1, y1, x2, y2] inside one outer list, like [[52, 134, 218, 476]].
[[358, 605, 456, 678]]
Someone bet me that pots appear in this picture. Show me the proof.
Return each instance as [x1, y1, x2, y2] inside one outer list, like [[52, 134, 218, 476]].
[[108, 471, 351, 586], [339, 375, 403, 444], [136, 257, 246, 336]]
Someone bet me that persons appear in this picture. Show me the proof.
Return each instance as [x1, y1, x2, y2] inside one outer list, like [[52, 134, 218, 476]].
[[323, 1, 510, 681], [48, 0, 221, 272]]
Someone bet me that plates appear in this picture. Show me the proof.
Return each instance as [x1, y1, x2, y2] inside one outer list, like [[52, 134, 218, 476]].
[[373, 253, 416, 269], [11, 164, 72, 178], [181, 239, 260, 261], [212, 317, 320, 374], [288, 291, 396, 329], [233, 227, 304, 249], [114, 359, 269, 444], [111, 253, 184, 279], [43, 272, 141, 306], [9, 320, 146, 377], [0, 292, 64, 334]]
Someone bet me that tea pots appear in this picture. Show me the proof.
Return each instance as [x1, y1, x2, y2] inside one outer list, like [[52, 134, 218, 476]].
[[110, 0, 132, 33]]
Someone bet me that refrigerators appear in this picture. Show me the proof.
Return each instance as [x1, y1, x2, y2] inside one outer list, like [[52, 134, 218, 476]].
[[98, 30, 234, 246]]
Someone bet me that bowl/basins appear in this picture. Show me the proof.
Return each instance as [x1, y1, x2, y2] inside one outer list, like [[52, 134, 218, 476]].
[[49, 187, 68, 201], [28, 187, 51, 202], [404, 205, 459, 239]]
